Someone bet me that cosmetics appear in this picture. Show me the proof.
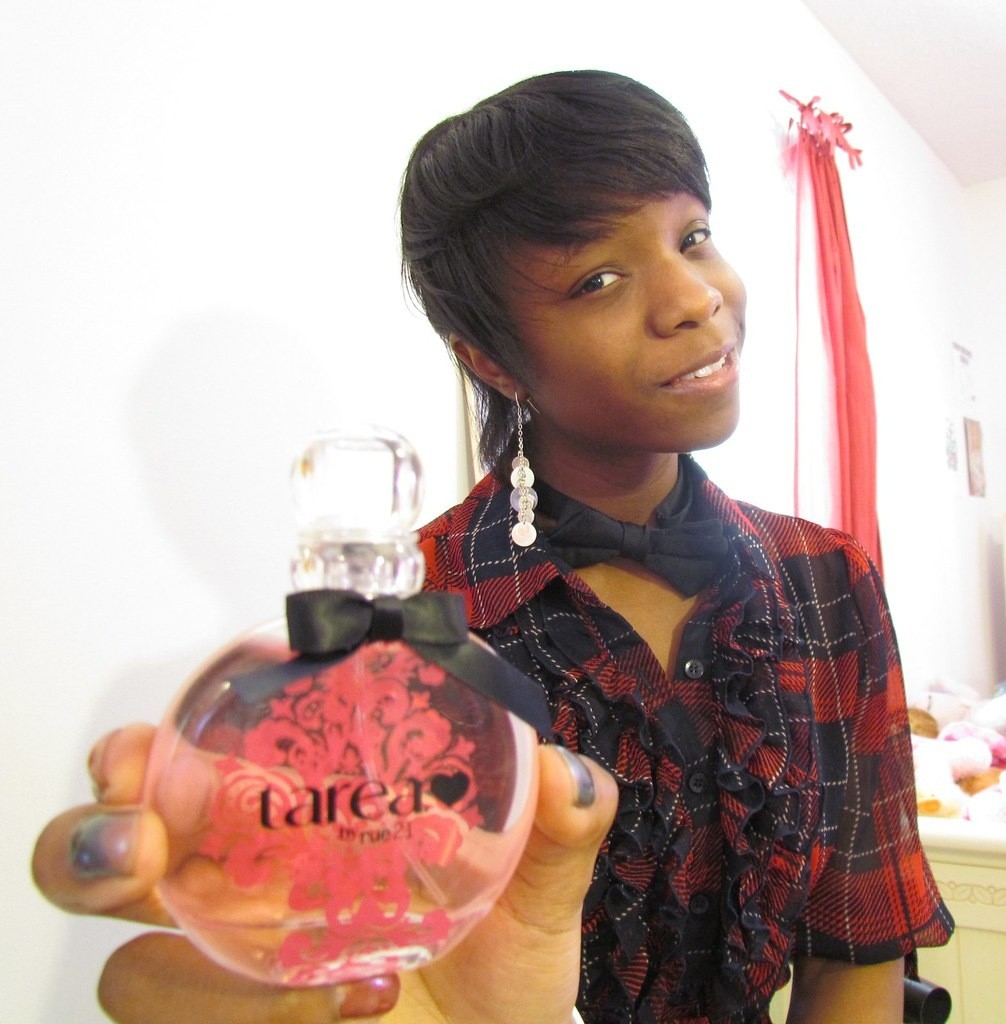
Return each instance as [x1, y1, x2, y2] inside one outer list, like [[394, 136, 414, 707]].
[[132, 430, 559, 985]]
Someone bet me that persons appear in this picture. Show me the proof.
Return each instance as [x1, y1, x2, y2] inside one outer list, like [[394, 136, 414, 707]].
[[32, 68, 956, 1024]]
